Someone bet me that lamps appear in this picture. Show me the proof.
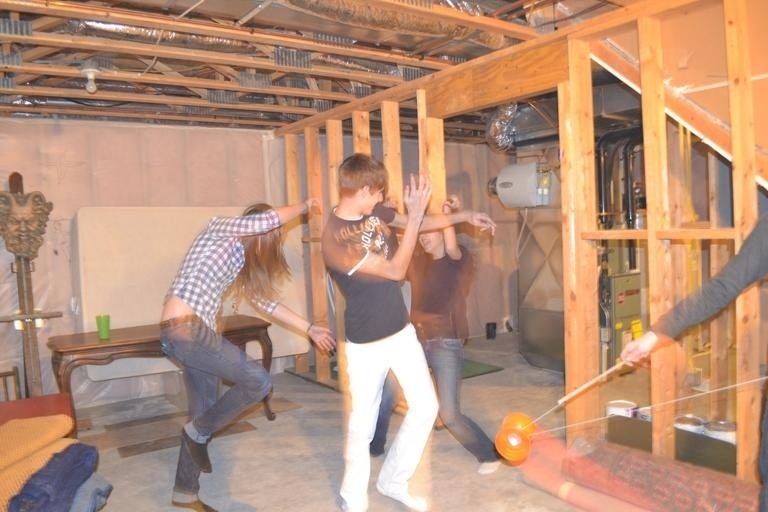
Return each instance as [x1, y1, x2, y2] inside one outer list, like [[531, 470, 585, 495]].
[[79, 59, 101, 93]]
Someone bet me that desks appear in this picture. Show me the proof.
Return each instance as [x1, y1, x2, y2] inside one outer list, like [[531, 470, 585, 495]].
[[46, 313, 274, 440]]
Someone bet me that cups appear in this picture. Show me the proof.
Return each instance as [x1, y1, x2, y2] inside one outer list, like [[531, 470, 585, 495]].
[[95, 314, 110, 341]]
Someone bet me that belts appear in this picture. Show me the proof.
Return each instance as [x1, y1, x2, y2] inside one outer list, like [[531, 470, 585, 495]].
[[159, 314, 201, 329]]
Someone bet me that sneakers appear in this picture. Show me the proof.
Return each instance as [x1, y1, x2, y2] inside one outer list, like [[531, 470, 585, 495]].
[[181, 426, 212, 473], [376, 482, 427, 511], [171, 499, 217, 512]]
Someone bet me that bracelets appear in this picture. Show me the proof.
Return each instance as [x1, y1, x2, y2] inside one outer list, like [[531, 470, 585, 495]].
[[305, 323, 313, 333], [442, 204, 451, 211], [301, 201, 308, 214]]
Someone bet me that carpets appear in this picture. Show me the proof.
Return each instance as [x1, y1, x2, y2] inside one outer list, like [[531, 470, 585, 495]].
[[309, 356, 504, 382], [103, 410, 257, 457]]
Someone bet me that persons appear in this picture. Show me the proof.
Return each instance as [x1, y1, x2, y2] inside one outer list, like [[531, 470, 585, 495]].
[[321, 152, 498, 512], [158, 197, 336, 512], [618, 213, 767, 512], [367, 195, 500, 475], [0, 191, 53, 259]]
